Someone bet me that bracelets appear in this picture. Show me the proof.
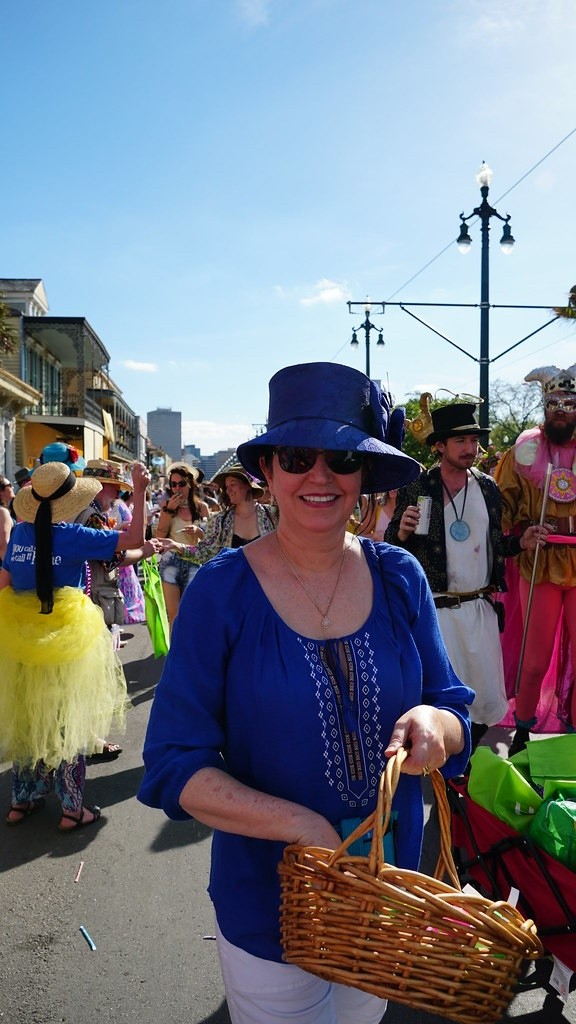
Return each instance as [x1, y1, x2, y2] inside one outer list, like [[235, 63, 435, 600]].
[[163, 506, 176, 518]]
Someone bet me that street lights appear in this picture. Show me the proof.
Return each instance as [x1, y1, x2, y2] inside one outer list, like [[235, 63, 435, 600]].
[[351, 295, 384, 379], [348, 159, 576, 470]]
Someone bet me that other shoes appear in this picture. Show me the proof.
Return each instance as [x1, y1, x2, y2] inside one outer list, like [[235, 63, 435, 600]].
[[91, 740, 123, 759]]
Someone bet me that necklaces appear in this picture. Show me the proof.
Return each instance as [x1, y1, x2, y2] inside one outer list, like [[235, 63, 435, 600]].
[[89, 498, 103, 514], [276, 530, 348, 629], [545, 438, 576, 503], [85, 561, 91, 598], [440, 472, 470, 542]]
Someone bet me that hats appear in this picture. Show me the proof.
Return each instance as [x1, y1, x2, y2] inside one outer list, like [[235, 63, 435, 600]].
[[236, 362, 424, 494], [426, 403, 492, 447], [14, 466, 31, 484], [524, 362, 576, 397], [28, 442, 86, 477], [212, 462, 264, 497], [82, 457, 134, 492], [12, 461, 104, 525]]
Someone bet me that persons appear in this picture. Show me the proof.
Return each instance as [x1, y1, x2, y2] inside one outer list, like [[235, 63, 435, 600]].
[[493, 363, 576, 757], [0, 442, 165, 831], [384, 404, 557, 776], [158, 462, 279, 631], [137, 362, 476, 1024]]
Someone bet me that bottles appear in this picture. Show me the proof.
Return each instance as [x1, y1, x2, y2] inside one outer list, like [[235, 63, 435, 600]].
[[200, 516, 208, 531]]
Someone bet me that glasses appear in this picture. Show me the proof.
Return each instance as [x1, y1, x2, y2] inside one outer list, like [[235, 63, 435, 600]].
[[272, 444, 362, 476], [3, 482, 12, 489], [168, 479, 188, 488]]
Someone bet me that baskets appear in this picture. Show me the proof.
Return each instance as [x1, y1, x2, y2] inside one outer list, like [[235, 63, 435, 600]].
[[279, 746, 542, 1024]]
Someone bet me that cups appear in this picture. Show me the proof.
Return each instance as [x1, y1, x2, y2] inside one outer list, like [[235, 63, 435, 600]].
[[108, 514, 116, 527]]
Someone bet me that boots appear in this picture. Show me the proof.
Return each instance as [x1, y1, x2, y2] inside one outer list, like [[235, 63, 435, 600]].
[[462, 721, 488, 776], [507, 712, 538, 757]]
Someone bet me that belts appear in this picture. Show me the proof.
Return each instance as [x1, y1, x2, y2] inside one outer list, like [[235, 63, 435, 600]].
[[433, 590, 493, 610]]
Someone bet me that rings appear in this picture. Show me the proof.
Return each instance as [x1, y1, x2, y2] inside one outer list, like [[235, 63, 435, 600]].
[[423, 765, 430, 777]]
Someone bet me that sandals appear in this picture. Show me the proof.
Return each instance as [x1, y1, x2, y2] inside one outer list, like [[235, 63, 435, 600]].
[[7, 796, 45, 824], [59, 804, 101, 832]]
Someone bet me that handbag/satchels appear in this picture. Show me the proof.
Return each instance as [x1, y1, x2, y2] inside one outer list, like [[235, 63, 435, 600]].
[[90, 585, 126, 624], [140, 553, 170, 660]]
